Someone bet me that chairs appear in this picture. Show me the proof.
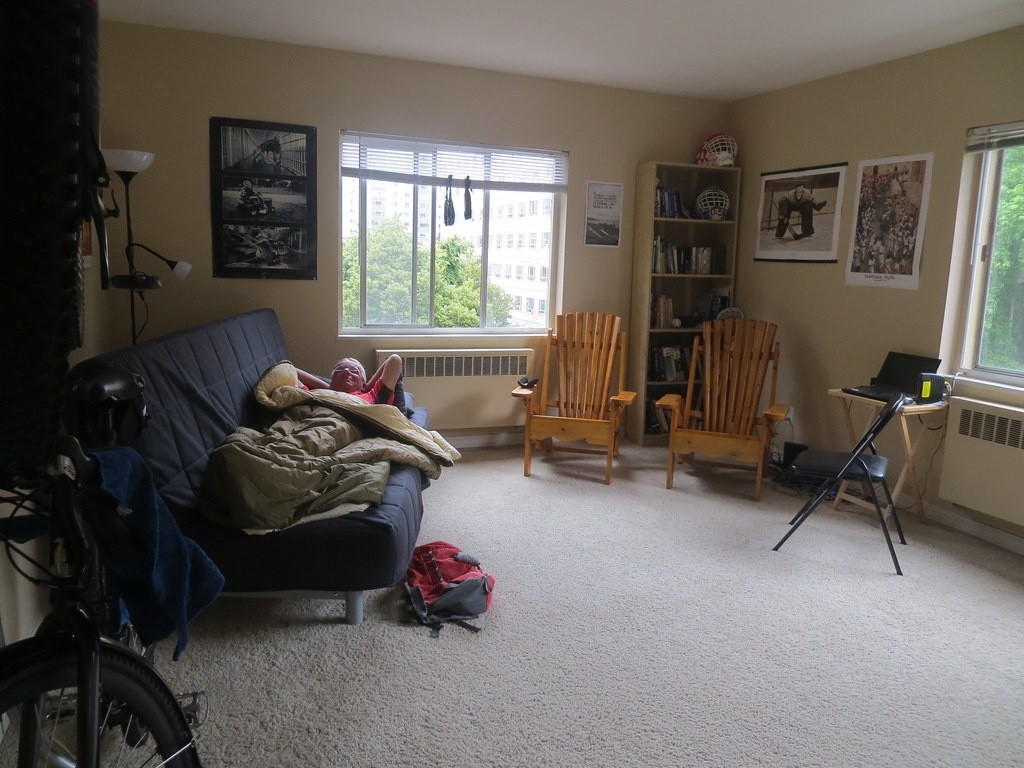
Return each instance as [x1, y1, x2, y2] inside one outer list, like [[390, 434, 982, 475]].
[[656, 318, 788, 502], [773, 391, 908, 576], [511, 311, 638, 485]]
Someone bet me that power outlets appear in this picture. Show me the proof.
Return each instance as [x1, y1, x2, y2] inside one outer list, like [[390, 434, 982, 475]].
[[784, 407, 794, 424]]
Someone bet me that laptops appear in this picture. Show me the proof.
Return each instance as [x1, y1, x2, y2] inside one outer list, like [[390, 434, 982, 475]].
[[841, 351, 943, 405]]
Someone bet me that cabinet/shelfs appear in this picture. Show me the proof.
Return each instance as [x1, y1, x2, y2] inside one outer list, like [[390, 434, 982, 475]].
[[625, 160, 741, 448]]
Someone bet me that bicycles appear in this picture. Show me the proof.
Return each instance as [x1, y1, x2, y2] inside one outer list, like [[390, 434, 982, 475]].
[[0, 354, 211, 768]]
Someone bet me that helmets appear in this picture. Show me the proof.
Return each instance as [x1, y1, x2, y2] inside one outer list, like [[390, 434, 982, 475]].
[[697, 133, 738, 167], [696, 185, 729, 221]]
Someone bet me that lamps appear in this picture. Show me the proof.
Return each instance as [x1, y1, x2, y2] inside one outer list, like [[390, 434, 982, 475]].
[[101, 148, 193, 346]]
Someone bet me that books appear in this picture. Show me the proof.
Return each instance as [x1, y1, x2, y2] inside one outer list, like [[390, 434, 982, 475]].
[[648, 339, 704, 381], [657, 186, 693, 218], [652, 235, 726, 274], [651, 285, 730, 327], [647, 397, 672, 434]]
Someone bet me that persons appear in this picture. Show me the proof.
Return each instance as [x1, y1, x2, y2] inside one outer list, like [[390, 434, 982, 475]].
[[854, 168, 918, 275], [776, 184, 826, 239], [218, 353, 403, 522], [240, 180, 258, 207]]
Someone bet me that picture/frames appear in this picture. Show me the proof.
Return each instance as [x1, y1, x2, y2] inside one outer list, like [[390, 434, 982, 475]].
[[209, 116, 317, 281]]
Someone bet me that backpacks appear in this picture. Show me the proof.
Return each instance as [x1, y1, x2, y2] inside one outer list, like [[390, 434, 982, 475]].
[[402, 541, 494, 625]]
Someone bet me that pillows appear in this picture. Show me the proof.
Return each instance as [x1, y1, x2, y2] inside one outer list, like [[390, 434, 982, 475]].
[[314, 374, 414, 417]]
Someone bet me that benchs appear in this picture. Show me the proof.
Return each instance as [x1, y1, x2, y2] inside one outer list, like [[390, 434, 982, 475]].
[[69, 307, 431, 624]]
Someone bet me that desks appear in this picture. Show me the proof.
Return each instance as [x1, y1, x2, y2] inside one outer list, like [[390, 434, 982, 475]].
[[828, 389, 948, 529]]
